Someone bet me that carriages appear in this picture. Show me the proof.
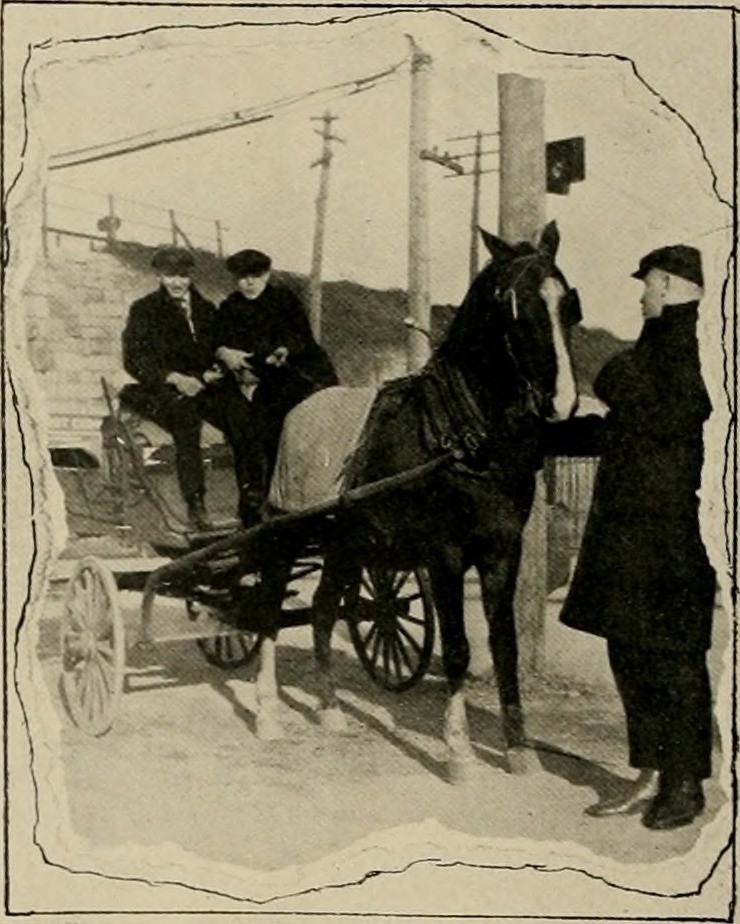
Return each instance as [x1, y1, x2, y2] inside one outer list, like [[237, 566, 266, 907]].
[[50, 217, 584, 783]]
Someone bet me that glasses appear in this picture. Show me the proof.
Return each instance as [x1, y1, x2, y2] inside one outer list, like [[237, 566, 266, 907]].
[[231, 269, 267, 278]]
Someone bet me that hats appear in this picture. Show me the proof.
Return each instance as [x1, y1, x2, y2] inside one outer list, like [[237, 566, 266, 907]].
[[632, 244, 703, 286], [225, 250, 270, 271], [152, 248, 196, 269]]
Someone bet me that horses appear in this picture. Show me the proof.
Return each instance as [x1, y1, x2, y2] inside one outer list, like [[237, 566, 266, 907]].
[[254, 215, 578, 785]]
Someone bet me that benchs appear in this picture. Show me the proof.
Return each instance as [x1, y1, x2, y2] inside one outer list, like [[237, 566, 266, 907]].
[[93, 364, 231, 531]]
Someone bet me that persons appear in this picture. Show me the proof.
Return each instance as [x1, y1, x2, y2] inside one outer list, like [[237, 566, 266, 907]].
[[524, 245, 717, 827], [121, 248, 266, 531], [214, 248, 340, 498]]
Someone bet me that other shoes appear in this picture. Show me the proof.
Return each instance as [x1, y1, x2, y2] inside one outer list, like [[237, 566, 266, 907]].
[[184, 494, 212, 531], [644, 778, 705, 831], [238, 489, 271, 524], [583, 767, 658, 817]]
